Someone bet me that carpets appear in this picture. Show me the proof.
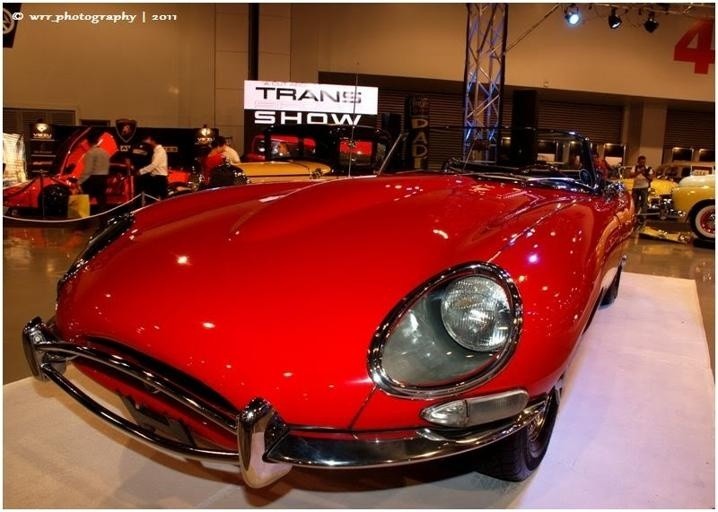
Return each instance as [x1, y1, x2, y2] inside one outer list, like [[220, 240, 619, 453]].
[[2, 268, 715, 508]]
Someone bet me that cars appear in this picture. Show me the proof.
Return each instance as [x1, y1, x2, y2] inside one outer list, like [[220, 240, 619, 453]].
[[612, 157, 714, 244]]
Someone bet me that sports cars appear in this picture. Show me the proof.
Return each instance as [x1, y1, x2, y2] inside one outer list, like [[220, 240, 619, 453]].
[[22, 124, 637, 494]]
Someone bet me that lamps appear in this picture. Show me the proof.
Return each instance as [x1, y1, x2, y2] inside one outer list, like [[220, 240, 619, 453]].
[[563, 3, 662, 34]]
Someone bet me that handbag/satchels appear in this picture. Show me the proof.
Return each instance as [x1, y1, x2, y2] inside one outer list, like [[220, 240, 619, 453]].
[[67, 183, 91, 219]]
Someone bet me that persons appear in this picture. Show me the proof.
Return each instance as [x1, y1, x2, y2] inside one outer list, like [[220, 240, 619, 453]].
[[216, 138, 241, 165], [202, 139, 225, 188], [78, 133, 109, 233], [631, 153, 656, 213], [136, 136, 170, 201]]
